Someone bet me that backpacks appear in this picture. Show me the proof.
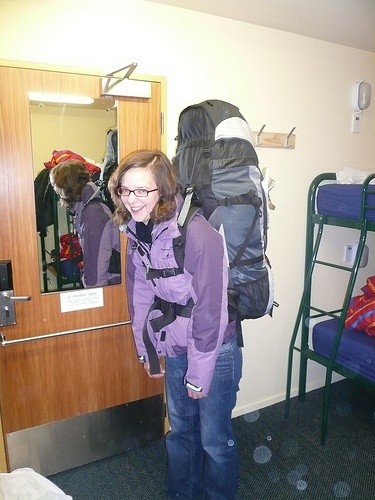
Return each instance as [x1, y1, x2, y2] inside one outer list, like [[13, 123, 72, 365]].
[[91, 127, 117, 209], [142, 97, 277, 375]]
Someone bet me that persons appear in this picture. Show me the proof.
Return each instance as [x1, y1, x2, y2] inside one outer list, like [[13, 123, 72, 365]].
[[49, 159, 122, 288], [108, 150, 242, 500]]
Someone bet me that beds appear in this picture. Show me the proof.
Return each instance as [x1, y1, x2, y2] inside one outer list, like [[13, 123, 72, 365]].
[[284, 171, 375, 444], [40, 169, 84, 292]]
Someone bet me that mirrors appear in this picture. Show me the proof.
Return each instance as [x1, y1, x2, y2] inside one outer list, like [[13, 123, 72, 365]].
[[27, 91, 124, 297]]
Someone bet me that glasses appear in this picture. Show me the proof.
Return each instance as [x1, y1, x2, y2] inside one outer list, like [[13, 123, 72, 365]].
[[117, 187, 158, 198]]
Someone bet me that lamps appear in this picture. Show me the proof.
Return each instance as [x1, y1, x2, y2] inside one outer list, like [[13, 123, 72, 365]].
[[351, 80, 371, 133]]
